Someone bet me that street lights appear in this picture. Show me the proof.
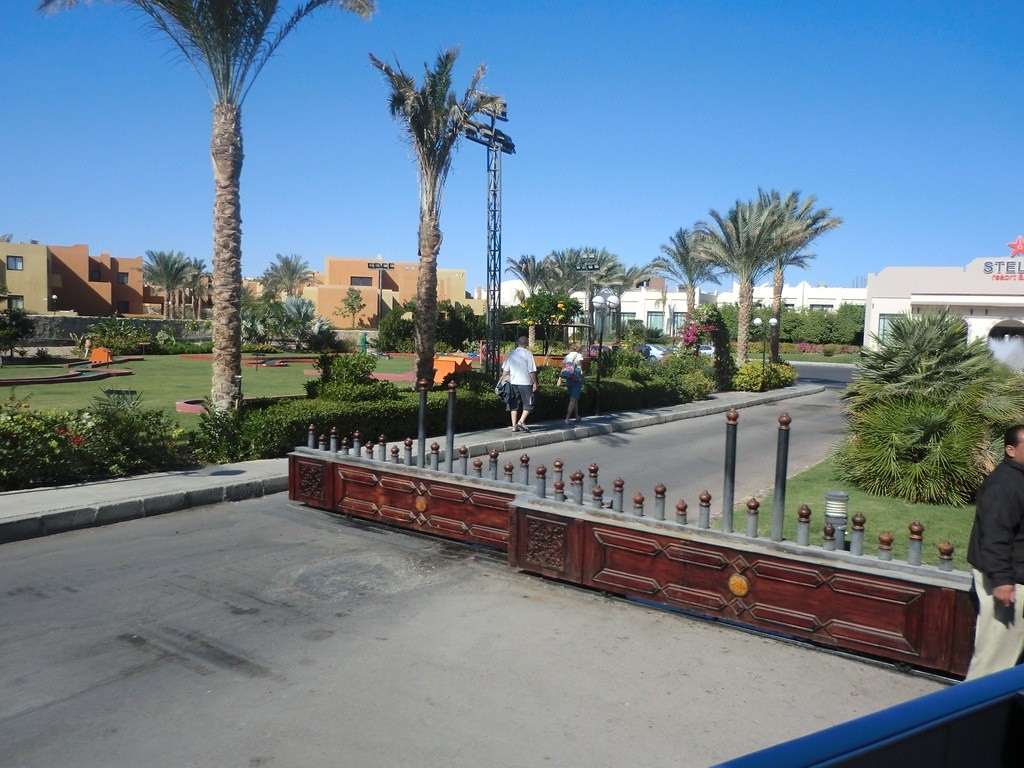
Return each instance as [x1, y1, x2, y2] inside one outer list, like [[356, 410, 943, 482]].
[[52, 295, 57, 314], [753, 317, 778, 392], [574, 262, 600, 356], [368, 262, 395, 332], [591, 295, 620, 415]]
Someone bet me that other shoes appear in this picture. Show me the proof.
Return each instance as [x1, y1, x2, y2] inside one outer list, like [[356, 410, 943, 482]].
[[564, 420, 573, 424], [576, 417, 581, 422]]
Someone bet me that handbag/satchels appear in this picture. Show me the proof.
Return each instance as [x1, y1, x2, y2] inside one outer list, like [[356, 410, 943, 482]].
[[559, 353, 581, 383]]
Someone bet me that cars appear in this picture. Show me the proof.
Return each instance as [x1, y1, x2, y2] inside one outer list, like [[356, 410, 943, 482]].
[[666, 344, 715, 357], [644, 343, 670, 362], [577, 344, 614, 358]]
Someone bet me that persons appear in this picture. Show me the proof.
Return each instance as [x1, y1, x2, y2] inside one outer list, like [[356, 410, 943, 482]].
[[497, 336, 537, 432], [469, 351, 476, 357], [965, 424, 1024, 680], [693, 345, 700, 355], [556, 342, 584, 423]]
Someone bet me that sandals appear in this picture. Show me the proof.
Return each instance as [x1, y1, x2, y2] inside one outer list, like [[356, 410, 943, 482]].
[[517, 420, 531, 433], [512, 426, 520, 432]]
